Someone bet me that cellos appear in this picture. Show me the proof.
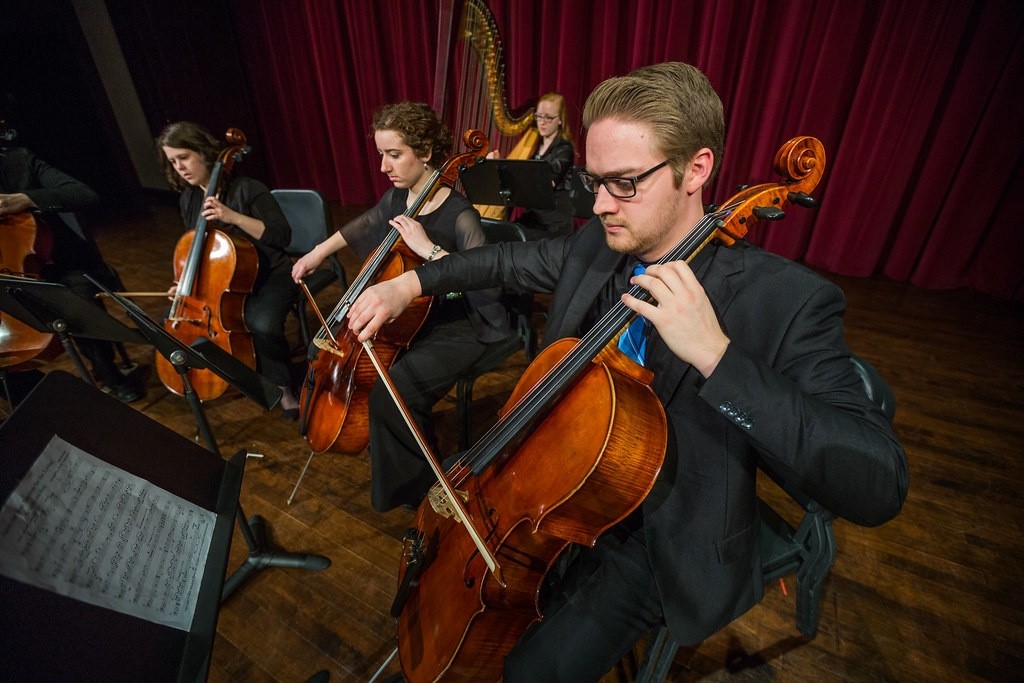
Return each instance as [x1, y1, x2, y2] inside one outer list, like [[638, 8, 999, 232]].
[[154, 126, 261, 406], [294, 125, 490, 459], [0, 209, 65, 376], [390, 135, 826, 683]]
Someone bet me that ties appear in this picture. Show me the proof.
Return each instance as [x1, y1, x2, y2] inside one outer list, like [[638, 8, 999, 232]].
[[619, 266, 653, 368]]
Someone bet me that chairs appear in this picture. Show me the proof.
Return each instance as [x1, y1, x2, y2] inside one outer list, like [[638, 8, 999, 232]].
[[270, 188, 348, 358], [616, 349, 897, 683], [564, 167, 595, 218], [58, 211, 135, 370], [442, 218, 537, 452]]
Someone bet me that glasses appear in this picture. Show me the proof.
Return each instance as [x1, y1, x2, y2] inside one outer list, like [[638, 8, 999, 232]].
[[532, 113, 560, 123], [576, 159, 671, 198]]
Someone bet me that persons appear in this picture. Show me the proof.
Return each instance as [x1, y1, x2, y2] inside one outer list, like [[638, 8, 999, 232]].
[[150, 121, 312, 414], [0, 120, 147, 404], [292, 59, 911, 682]]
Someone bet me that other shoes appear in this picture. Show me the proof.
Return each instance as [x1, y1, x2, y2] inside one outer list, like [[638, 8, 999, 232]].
[[281, 389, 301, 421]]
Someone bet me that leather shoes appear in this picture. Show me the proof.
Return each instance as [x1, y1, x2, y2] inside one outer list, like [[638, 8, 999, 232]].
[[105, 368, 142, 403]]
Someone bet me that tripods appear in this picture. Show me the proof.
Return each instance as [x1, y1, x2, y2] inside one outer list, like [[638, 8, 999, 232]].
[[82, 273, 332, 605]]
